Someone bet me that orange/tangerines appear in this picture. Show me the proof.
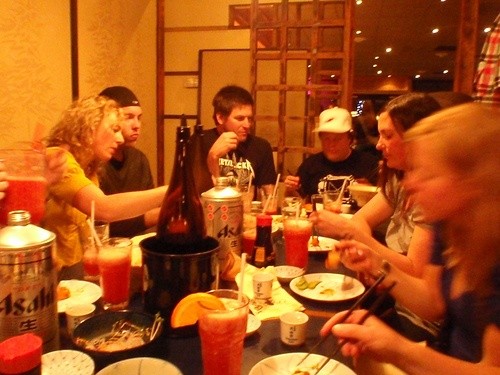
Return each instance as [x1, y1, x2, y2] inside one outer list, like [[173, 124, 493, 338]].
[[171, 292, 226, 328]]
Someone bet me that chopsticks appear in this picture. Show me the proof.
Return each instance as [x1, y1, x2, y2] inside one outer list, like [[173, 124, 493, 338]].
[[296, 274, 396, 374]]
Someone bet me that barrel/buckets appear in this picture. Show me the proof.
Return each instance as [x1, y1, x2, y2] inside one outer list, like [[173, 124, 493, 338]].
[[139, 235, 222, 338]]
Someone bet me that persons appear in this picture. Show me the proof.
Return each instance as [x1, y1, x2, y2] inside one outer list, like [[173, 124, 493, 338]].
[[0, 162, 9, 199], [310, 88, 500, 375], [1, 94, 171, 276], [284, 107, 383, 200], [185, 86, 277, 201], [92, 86, 161, 237]]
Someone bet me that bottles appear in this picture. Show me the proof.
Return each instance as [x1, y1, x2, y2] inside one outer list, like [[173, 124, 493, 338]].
[[1, 209, 61, 353], [158, 123, 276, 266], [0, 332, 43, 375]]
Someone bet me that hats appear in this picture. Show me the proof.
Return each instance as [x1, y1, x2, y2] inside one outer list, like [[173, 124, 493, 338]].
[[312, 107, 352, 133], [99, 86, 141, 107]]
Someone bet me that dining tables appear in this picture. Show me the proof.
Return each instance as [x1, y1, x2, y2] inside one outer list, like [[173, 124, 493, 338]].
[[56, 241, 383, 375]]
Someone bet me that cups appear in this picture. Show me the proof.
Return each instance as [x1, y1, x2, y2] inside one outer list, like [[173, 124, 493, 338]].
[[282, 218, 313, 271], [79, 219, 110, 282], [281, 196, 302, 217], [0, 148, 48, 225], [252, 272, 276, 303], [66, 303, 95, 335], [242, 214, 258, 256], [97, 237, 132, 311], [235, 183, 254, 213], [197, 288, 249, 374], [280, 311, 308, 346], [259, 186, 278, 215], [322, 190, 344, 212]]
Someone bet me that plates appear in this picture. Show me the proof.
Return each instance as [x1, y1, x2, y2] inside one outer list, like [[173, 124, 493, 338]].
[[275, 265, 307, 283], [40, 347, 95, 375], [95, 356, 183, 374], [69, 310, 162, 364], [249, 350, 358, 374], [128, 230, 156, 267], [55, 279, 103, 314], [244, 314, 261, 336], [289, 272, 366, 303], [242, 196, 354, 252]]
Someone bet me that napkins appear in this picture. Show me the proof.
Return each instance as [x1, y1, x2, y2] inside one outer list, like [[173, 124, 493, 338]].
[[235, 273, 306, 320]]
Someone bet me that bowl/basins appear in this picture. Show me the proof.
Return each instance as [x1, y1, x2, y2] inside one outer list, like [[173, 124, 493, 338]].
[[349, 185, 382, 206]]
